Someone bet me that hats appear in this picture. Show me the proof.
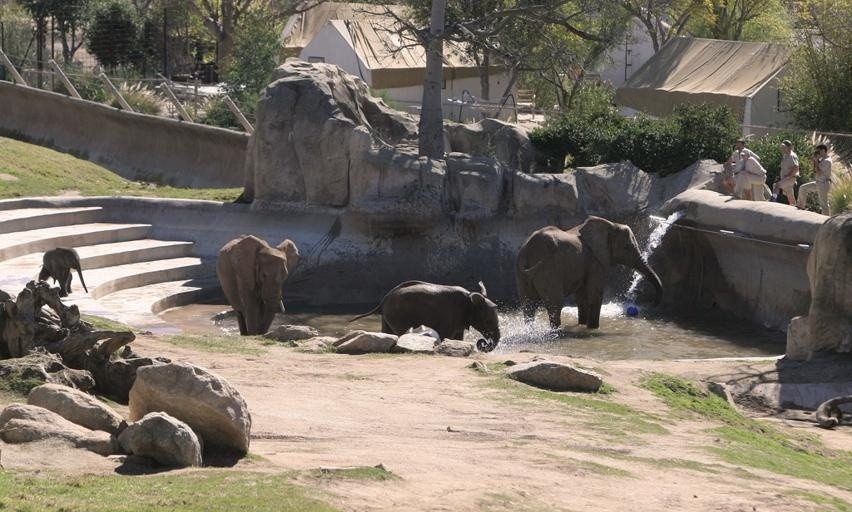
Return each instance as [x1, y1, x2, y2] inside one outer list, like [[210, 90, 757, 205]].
[[781, 140, 792, 147], [740, 150, 750, 156]]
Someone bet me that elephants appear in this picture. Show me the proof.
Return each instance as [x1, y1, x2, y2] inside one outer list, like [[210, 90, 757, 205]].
[[515, 215, 664, 330], [39, 247, 88, 296], [216, 233, 299, 336], [348, 279, 501, 353]]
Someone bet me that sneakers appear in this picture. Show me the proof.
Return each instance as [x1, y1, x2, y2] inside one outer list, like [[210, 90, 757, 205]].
[[766, 196, 777, 202]]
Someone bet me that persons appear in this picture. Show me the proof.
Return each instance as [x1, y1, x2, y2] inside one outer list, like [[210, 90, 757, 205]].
[[733, 150, 767, 201], [724, 138, 761, 200], [795, 145, 832, 216], [768, 140, 800, 205]]
